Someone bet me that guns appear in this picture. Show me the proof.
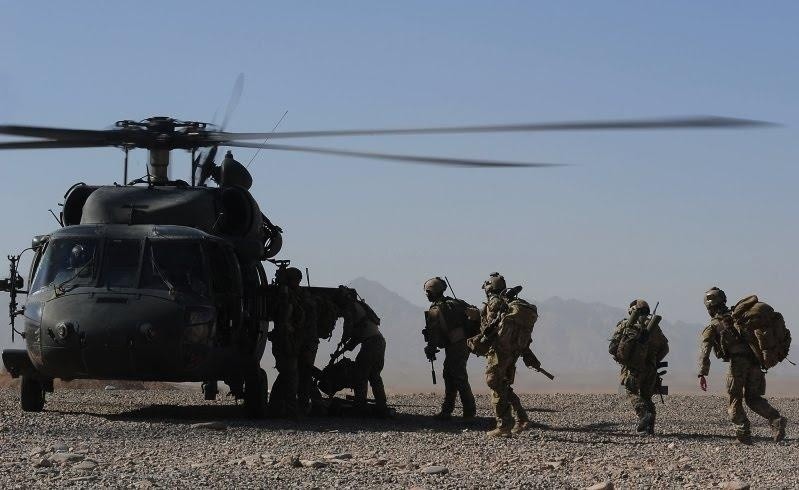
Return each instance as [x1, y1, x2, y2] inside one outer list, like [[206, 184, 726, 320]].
[[656, 360, 668, 404]]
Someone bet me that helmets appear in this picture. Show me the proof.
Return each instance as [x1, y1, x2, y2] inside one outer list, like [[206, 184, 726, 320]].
[[704, 288, 726, 307], [629, 300, 650, 315], [424, 277, 447, 298], [286, 268, 302, 280], [484, 272, 506, 293]]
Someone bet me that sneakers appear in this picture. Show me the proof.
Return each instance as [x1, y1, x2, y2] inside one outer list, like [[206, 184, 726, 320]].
[[464, 413, 473, 423], [512, 420, 528, 434], [434, 410, 450, 419], [487, 428, 511, 438], [770, 416, 787, 442], [638, 414, 654, 434], [733, 437, 751, 444]]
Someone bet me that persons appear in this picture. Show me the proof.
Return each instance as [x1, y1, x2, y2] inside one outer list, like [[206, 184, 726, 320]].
[[473, 272, 533, 437], [55, 245, 89, 287], [421, 277, 480, 421], [331, 287, 388, 419], [269, 269, 320, 417], [608, 299, 669, 436], [696, 287, 788, 447]]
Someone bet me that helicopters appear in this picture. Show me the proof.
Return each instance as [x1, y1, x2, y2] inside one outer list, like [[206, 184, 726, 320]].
[[0, 71, 794, 415]]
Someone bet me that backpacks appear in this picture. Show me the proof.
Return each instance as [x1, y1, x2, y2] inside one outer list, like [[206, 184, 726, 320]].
[[430, 297, 481, 339], [487, 294, 538, 355], [615, 321, 648, 371]]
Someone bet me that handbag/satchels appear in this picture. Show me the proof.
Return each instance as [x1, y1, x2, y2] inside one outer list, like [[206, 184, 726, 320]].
[[319, 358, 355, 394]]
